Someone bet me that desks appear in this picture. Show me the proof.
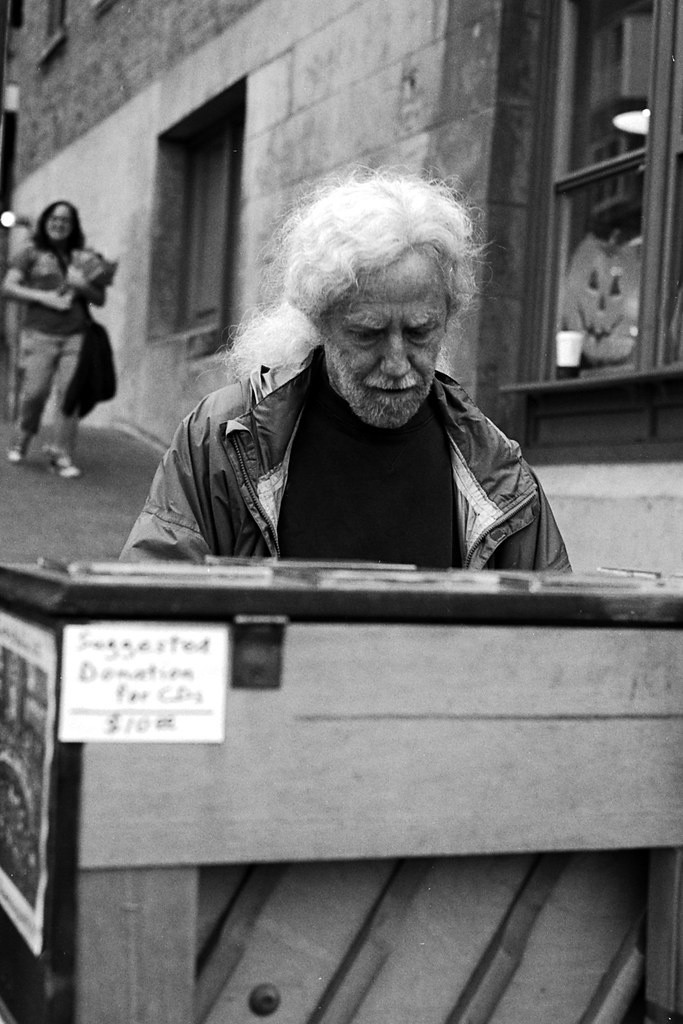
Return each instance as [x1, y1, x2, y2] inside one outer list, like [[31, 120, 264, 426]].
[[0, 558, 683, 1024]]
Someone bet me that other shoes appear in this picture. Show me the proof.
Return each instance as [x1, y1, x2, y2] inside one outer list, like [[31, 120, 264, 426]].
[[7, 449, 25, 463], [46, 455, 81, 477]]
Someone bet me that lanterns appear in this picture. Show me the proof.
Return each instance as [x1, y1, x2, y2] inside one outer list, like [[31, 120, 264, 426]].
[[564, 227, 641, 363]]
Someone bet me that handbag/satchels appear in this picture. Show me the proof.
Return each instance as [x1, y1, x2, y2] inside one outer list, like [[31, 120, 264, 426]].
[[62, 321, 116, 418]]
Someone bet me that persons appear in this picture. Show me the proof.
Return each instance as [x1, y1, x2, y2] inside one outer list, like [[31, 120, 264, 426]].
[[117, 173, 572, 569], [0, 201, 106, 477]]
